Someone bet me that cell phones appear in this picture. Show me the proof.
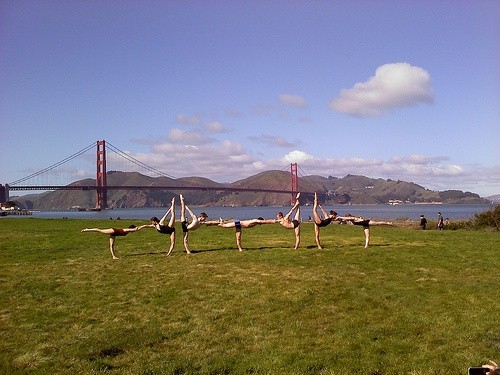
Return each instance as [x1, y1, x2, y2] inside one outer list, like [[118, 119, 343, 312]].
[[468, 367, 490, 375]]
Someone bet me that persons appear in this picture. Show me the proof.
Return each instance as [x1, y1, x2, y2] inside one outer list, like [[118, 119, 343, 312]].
[[143, 197, 175, 255], [218, 192, 301, 251], [437, 212, 443, 230], [336, 214, 393, 248], [179, 194, 222, 254], [80, 225, 146, 259], [313, 191, 342, 249], [420, 215, 427, 230], [444, 217, 449, 230]]
